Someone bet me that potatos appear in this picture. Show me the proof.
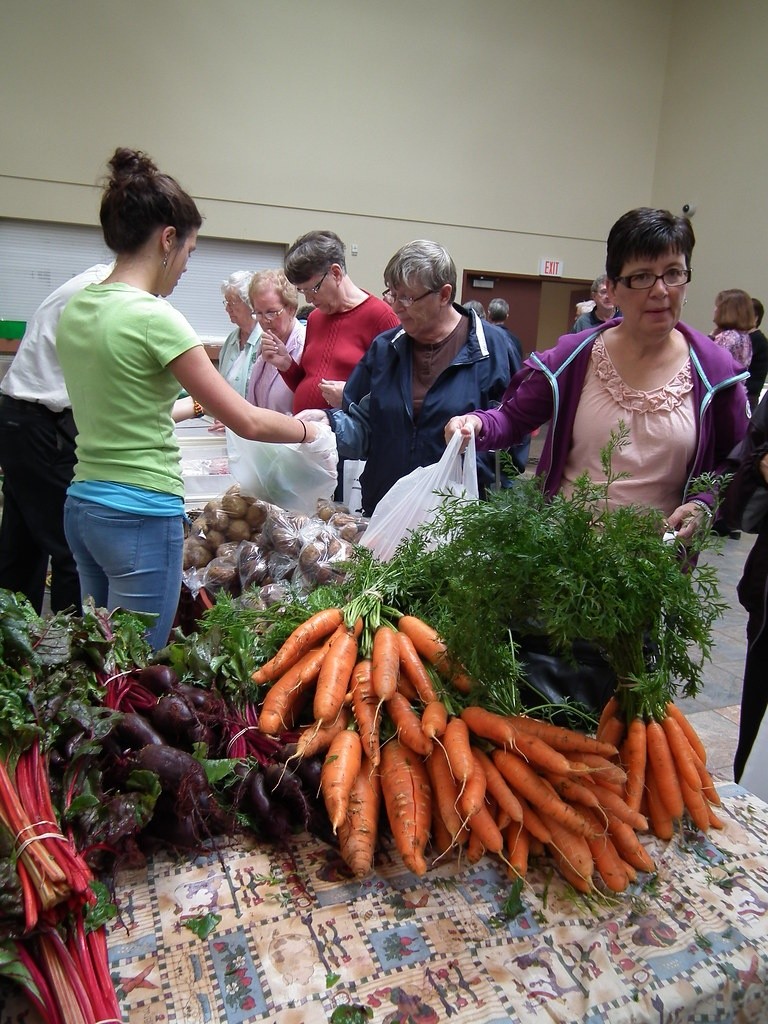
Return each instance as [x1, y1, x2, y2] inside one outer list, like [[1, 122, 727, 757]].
[[180, 483, 371, 627]]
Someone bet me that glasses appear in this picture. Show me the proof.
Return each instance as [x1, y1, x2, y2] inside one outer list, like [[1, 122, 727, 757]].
[[382, 289, 433, 307], [614, 268, 692, 290], [297, 261, 340, 295], [250, 304, 287, 322], [223, 301, 244, 309]]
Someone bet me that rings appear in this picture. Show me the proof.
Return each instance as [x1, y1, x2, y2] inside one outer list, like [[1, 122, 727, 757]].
[[327, 400, 330, 405]]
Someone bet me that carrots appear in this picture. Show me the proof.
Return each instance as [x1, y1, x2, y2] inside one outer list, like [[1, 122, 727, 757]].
[[247, 606, 726, 897]]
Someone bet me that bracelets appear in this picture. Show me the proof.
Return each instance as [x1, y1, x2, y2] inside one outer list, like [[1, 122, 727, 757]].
[[194, 400, 204, 418], [688, 499, 712, 524], [299, 419, 306, 442], [708, 335, 715, 341]]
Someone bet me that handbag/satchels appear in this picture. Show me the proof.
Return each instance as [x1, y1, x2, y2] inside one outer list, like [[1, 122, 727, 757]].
[[342, 423, 479, 585], [224, 420, 339, 518]]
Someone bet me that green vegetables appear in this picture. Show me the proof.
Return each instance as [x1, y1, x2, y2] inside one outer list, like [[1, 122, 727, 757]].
[[0, 583, 164, 1024]]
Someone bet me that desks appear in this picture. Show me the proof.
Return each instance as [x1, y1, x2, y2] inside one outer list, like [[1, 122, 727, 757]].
[[96, 766, 768, 1024]]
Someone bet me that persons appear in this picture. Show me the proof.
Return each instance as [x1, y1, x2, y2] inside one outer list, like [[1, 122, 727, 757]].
[[205, 270, 264, 433], [260, 230, 400, 505], [733, 391, 768, 783], [293, 241, 531, 517], [56, 148, 320, 653], [706, 289, 768, 540], [575, 272, 622, 334], [445, 208, 751, 671], [486, 298, 522, 358], [296, 305, 315, 327], [463, 300, 485, 319], [0, 260, 213, 620], [246, 269, 305, 416]]
